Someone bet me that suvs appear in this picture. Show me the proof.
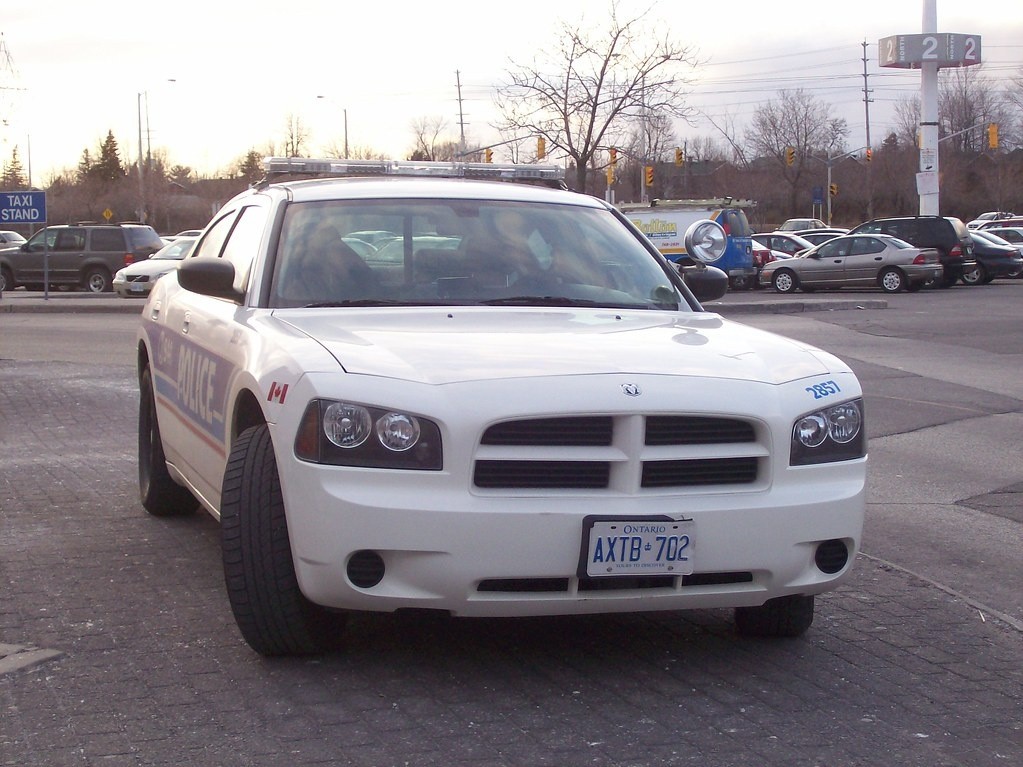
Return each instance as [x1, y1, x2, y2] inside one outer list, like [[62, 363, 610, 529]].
[[0, 220, 165, 293], [966, 211, 1015, 231], [824, 215, 978, 289]]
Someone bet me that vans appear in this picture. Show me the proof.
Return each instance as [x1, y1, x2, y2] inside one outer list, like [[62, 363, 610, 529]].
[[612, 196, 758, 280]]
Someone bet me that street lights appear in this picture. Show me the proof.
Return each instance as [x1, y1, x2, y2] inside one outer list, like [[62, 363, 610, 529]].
[[316, 94, 349, 160], [139, 79, 176, 200], [785, 145, 872, 227]]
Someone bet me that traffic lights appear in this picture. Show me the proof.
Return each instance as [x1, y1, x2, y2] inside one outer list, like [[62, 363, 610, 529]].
[[830, 183, 838, 196], [610, 148, 618, 166], [676, 150, 683, 167], [646, 166, 653, 187], [485, 148, 493, 163], [866, 149, 872, 162], [786, 147, 795, 167]]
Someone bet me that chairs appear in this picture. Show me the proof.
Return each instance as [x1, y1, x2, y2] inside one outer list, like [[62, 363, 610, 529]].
[[773, 239, 786, 251], [282, 211, 385, 300], [59, 236, 76, 250], [436, 237, 545, 295]]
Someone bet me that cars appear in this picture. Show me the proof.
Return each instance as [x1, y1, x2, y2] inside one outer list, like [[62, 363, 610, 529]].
[[159, 230, 208, 256], [729, 218, 897, 291], [959, 233, 1023, 285], [974, 216, 1023, 279], [0, 230, 29, 249], [759, 234, 944, 294], [339, 230, 462, 266], [112, 237, 200, 298], [47, 234, 83, 247], [141, 160, 868, 662]]
